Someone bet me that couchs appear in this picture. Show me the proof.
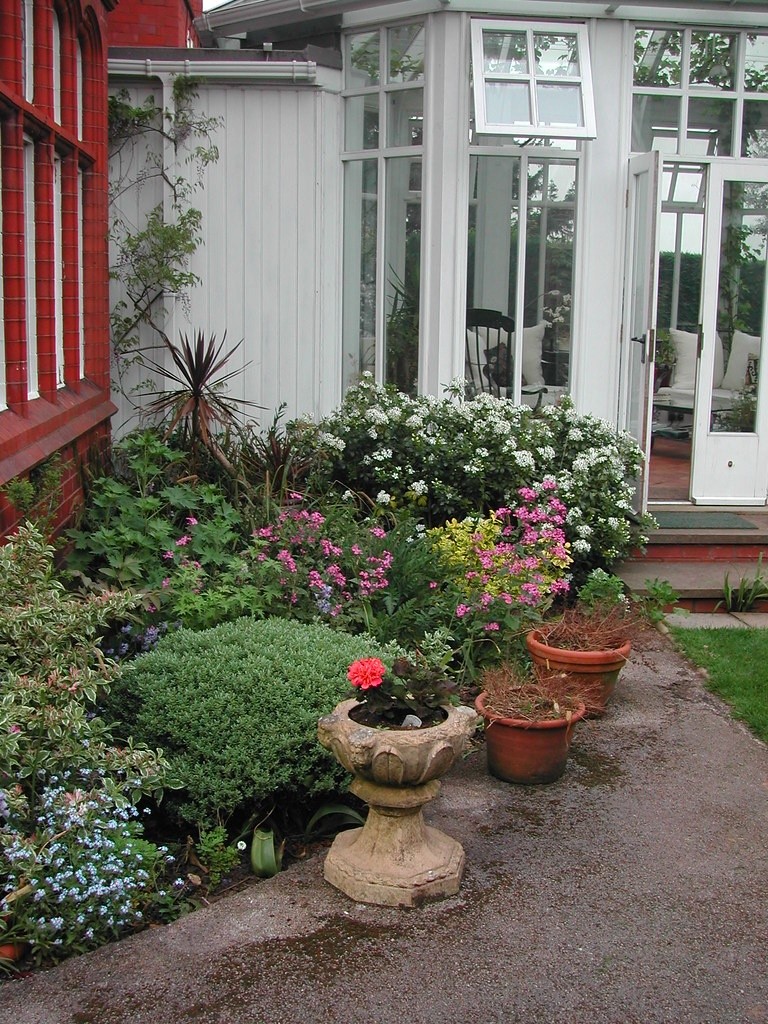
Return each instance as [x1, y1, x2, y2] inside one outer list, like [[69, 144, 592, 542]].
[[465, 323, 569, 409], [656, 328, 764, 421]]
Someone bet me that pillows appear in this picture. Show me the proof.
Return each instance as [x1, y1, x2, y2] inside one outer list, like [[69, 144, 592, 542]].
[[466, 329, 491, 392], [669, 327, 724, 389], [721, 330, 761, 392], [473, 321, 546, 385]]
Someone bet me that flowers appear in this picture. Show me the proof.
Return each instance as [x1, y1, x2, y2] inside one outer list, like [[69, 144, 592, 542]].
[[346, 629, 465, 728]]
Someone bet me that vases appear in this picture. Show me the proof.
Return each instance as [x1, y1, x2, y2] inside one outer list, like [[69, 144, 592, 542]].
[[316, 697, 478, 909]]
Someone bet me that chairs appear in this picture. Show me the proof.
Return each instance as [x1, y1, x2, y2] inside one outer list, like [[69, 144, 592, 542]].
[[464, 308, 548, 414]]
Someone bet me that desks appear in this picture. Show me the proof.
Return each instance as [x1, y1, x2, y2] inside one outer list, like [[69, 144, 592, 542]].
[[651, 390, 748, 451]]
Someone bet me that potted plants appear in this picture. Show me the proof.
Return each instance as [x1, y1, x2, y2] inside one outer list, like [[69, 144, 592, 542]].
[[526, 604, 655, 713], [654, 327, 678, 396], [474, 662, 607, 785]]
